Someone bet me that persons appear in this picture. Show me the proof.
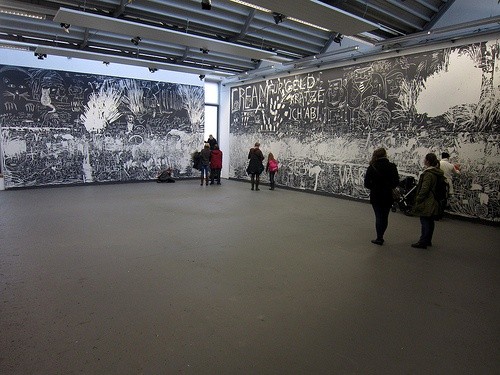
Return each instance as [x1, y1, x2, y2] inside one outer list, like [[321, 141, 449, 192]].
[[265, 153, 279, 191], [410, 153, 446, 250], [210, 144, 222, 186], [439, 152, 462, 196], [193, 144, 211, 186], [204, 134, 218, 151], [246, 142, 264, 191], [364, 148, 400, 244]]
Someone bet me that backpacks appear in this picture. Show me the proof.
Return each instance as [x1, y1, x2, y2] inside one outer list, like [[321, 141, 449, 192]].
[[426, 171, 450, 208], [193, 152, 201, 171], [269, 163, 278, 171]]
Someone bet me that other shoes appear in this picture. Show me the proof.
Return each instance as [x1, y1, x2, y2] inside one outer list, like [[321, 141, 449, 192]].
[[201, 184, 203, 187], [217, 182, 220, 184], [372, 238, 385, 246], [206, 182, 208, 186], [210, 182, 214, 185], [411, 240, 433, 249], [255, 182, 261, 191], [251, 183, 254, 190]]
